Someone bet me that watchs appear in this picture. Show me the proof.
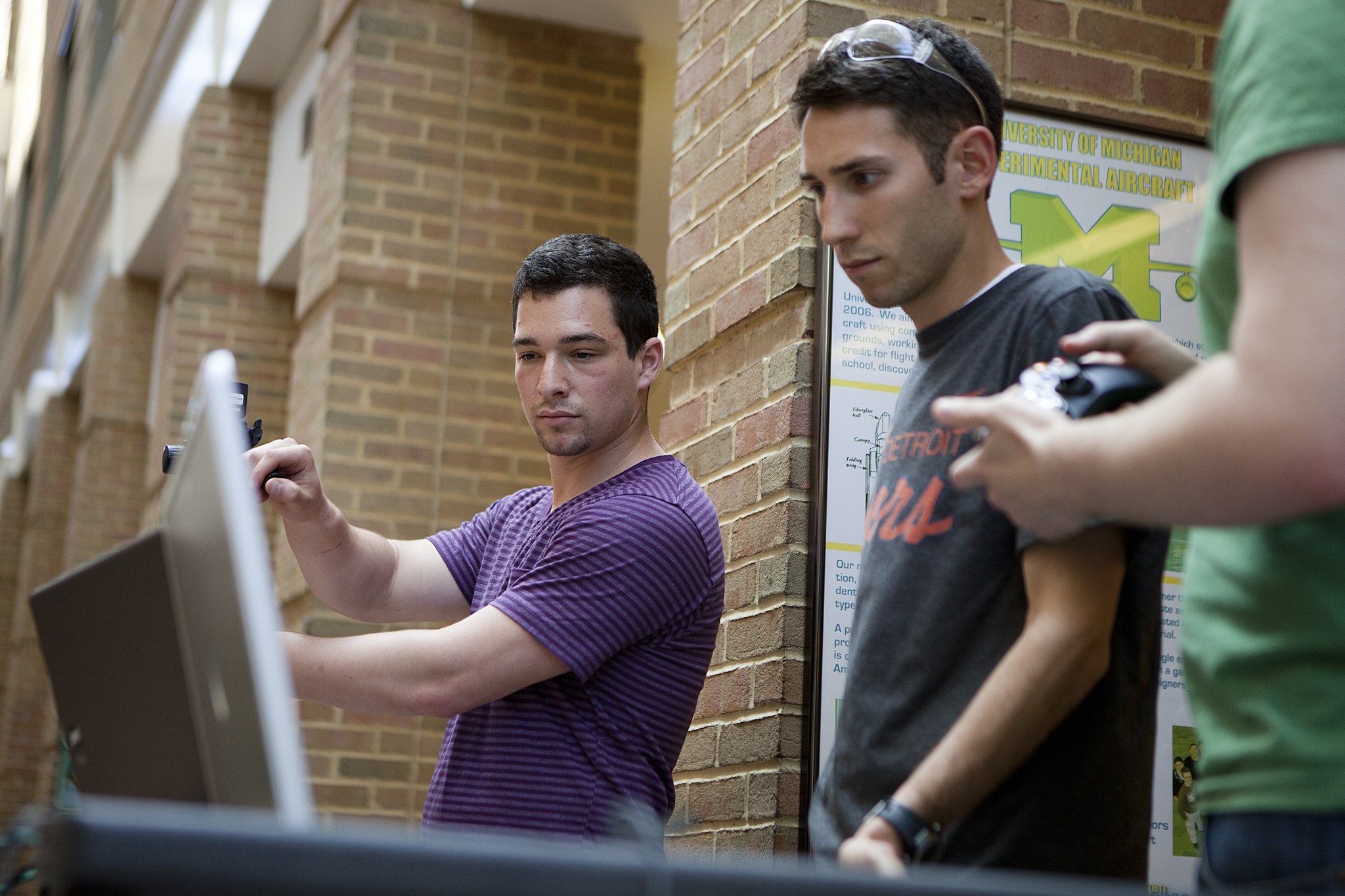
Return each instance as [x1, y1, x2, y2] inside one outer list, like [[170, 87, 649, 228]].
[[867, 797, 942, 867]]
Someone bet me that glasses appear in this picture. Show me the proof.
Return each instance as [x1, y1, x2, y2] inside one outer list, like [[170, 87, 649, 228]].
[[816, 19, 992, 133]]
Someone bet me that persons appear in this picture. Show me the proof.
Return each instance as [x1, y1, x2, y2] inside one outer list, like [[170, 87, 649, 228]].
[[241, 233, 725, 843], [794, 19, 1172, 880], [933, 1, 1345, 896]]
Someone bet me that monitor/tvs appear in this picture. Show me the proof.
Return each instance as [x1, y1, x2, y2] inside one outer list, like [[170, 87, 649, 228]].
[[155, 338, 332, 830], [23, 530, 210, 806]]
